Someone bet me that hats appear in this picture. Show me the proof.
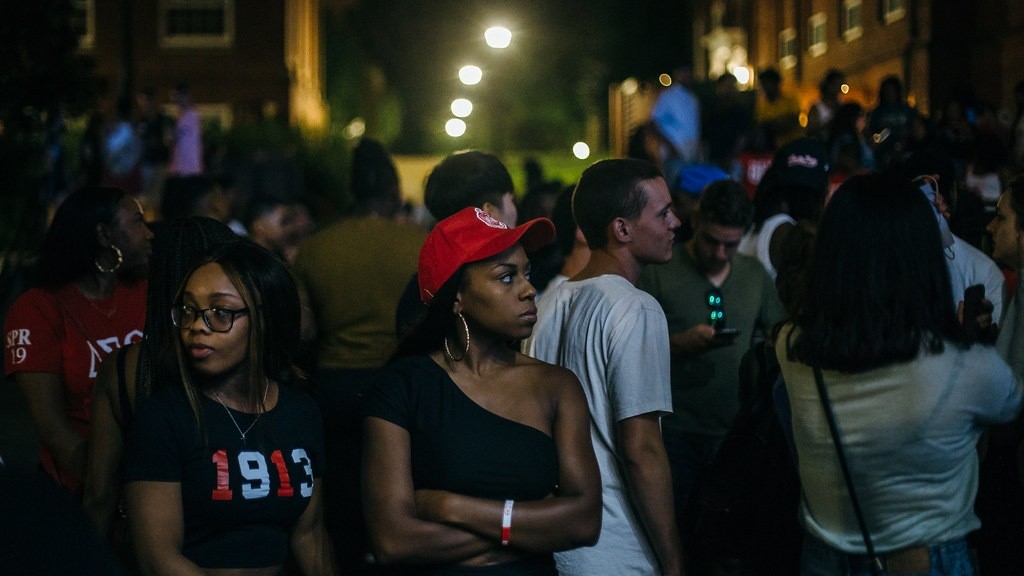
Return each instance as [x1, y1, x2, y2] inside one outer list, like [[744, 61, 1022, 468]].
[[417, 207, 556, 303]]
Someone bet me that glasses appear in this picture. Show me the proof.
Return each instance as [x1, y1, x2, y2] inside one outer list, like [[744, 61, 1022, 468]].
[[170, 304, 248, 332], [706, 288, 727, 327]]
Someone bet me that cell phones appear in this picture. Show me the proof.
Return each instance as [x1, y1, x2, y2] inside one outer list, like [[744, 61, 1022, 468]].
[[714, 328, 743, 342], [962, 282, 985, 328]]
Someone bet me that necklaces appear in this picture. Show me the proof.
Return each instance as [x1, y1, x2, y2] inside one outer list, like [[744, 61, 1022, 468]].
[[209, 375, 270, 446]]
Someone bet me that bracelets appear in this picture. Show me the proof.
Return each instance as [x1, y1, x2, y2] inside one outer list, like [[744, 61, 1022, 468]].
[[502, 499, 515, 546]]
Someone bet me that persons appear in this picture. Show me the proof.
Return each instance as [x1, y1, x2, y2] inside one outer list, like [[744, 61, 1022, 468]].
[[620, 57, 1024, 206], [395, 152, 518, 356], [0, 76, 580, 251], [773, 170, 1024, 576], [6, 186, 154, 497], [636, 180, 790, 517], [83, 216, 244, 558], [987, 176, 1024, 387], [362, 206, 604, 576], [295, 137, 431, 369], [518, 159, 684, 576], [120, 241, 345, 576]]
